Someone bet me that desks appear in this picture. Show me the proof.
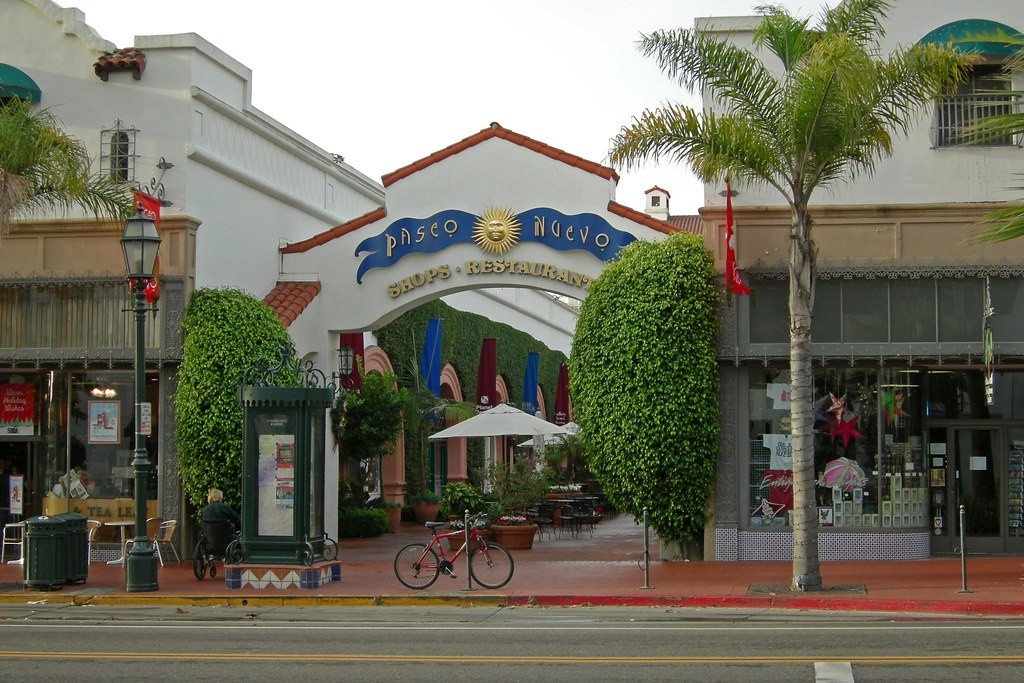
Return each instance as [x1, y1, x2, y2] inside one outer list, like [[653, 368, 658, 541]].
[[105, 519, 136, 568], [6, 524, 24, 566], [575, 497, 600, 531]]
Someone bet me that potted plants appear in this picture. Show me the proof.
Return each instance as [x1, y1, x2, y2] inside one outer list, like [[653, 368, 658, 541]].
[[410, 489, 438, 523], [385, 502, 401, 533]]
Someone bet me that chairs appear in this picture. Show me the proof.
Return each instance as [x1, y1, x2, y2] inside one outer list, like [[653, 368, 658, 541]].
[[1, 521, 25, 563], [122, 517, 164, 567], [528, 502, 594, 541], [155, 520, 180, 563], [86, 520, 101, 564]]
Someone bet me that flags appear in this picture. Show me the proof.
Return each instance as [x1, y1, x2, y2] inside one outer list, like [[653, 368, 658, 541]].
[[725, 183, 751, 295]]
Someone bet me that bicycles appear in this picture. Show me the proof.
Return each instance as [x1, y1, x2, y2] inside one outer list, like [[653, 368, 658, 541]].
[[393, 511, 515, 590]]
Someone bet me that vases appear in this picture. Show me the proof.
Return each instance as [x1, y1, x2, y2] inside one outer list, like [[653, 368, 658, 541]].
[[546, 492, 584, 523], [445, 529, 490, 549], [491, 524, 537, 548]]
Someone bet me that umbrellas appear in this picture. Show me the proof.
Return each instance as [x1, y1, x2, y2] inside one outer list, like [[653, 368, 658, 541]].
[[822, 457, 865, 492], [517, 421, 583, 447], [428, 400, 567, 463], [533, 407, 545, 462]]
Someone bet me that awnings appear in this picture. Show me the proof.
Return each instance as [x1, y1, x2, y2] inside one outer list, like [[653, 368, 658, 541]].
[[0, 63, 41, 102], [915, 18, 1024, 57]]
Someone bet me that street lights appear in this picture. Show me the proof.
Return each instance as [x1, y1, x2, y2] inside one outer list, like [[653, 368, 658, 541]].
[[118, 201, 161, 592]]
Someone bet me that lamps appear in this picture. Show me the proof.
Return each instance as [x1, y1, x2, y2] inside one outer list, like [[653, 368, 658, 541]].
[[90, 377, 118, 398]]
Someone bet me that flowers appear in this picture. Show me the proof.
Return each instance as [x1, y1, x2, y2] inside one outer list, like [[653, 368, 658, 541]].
[[548, 484, 581, 493], [496, 513, 534, 525], [449, 520, 486, 529]]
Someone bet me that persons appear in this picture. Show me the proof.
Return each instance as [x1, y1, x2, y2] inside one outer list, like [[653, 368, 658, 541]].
[[202, 489, 240, 530], [0, 459, 27, 550], [59, 465, 95, 499]]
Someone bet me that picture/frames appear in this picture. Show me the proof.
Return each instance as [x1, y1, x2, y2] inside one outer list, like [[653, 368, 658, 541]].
[[88, 400, 121, 444], [818, 476, 927, 529]]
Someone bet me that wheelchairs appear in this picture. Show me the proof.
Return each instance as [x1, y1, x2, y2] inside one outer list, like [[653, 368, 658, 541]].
[[192, 519, 241, 581]]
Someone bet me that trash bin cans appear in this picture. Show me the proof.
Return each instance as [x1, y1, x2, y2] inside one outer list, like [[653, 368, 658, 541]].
[[23, 515, 66, 587], [53, 512, 89, 584]]
